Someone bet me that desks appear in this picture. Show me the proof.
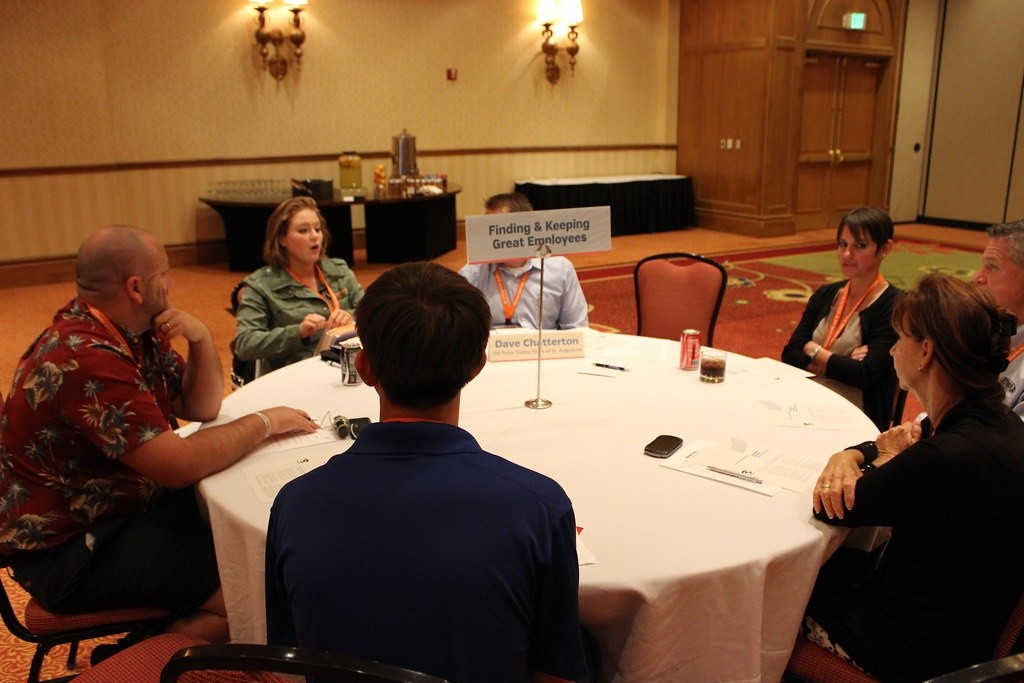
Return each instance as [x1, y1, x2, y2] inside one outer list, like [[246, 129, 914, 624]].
[[199, 183, 462, 273], [514, 174, 697, 238], [173, 332, 893, 682]]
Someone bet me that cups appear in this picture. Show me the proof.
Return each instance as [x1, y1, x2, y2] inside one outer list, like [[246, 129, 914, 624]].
[[700, 351, 727, 383], [388, 176, 444, 193]]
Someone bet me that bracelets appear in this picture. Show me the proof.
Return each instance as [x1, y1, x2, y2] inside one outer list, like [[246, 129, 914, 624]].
[[254, 412, 271, 436], [809, 344, 821, 359]]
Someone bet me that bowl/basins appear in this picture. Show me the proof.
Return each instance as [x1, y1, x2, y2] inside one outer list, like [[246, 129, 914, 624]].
[[292, 179, 333, 202]]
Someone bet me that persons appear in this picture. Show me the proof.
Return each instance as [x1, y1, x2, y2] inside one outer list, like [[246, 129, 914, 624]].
[[0, 224, 318, 666], [458, 192, 589, 331], [781, 204, 907, 433], [265, 259, 600, 683], [799, 272, 1024, 683], [233, 196, 366, 380], [969, 219, 1024, 422]]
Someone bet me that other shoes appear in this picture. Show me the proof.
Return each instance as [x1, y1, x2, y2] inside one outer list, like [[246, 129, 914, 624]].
[[90, 643, 118, 668]]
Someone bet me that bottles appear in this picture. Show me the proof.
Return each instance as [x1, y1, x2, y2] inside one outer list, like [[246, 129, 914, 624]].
[[339, 151, 362, 188], [373, 163, 386, 189]]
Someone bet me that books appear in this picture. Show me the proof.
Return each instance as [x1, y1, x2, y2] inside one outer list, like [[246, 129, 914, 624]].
[[313, 323, 358, 356]]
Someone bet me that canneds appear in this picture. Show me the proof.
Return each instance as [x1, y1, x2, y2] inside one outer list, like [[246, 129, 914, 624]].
[[339, 342, 363, 385], [680, 329, 701, 370]]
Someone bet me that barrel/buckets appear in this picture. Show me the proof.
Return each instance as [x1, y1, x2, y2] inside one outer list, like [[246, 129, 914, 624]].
[[392, 128, 416, 179]]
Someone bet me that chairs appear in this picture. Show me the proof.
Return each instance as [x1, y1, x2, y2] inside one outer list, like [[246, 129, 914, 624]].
[[70, 632, 213, 682], [160, 645, 450, 683], [0, 394, 173, 683], [633, 252, 728, 348], [786, 594, 1024, 683]]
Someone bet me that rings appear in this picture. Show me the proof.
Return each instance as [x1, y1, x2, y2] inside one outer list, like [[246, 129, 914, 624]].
[[821, 485, 830, 488], [166, 323, 172, 330]]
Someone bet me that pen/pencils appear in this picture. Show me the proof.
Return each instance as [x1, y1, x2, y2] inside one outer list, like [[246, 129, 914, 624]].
[[311, 418, 319, 421], [593, 362, 626, 370], [327, 360, 342, 367], [704, 463, 764, 485]]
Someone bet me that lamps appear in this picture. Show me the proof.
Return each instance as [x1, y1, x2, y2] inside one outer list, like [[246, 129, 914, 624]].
[[251, 0, 308, 82], [537, 0, 583, 85]]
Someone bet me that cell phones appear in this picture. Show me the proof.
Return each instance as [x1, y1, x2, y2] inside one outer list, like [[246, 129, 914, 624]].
[[348, 417, 372, 440], [645, 435, 683, 457]]
